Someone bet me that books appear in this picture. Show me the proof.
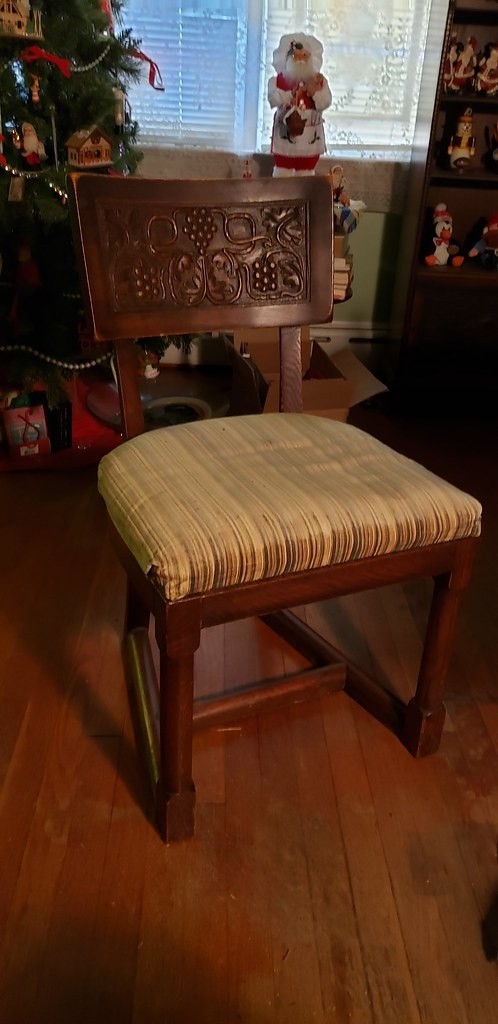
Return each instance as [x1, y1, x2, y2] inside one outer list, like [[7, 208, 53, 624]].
[[333, 245, 356, 302]]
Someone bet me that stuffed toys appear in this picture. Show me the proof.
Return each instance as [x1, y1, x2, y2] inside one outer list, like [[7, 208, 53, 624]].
[[468, 216, 498, 270], [422, 202, 465, 268]]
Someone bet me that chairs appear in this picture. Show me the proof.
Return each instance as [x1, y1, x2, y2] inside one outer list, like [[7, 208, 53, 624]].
[[66, 169, 484, 848]]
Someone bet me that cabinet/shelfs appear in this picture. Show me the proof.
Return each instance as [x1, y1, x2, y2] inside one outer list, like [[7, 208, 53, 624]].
[[381, 0, 497, 382]]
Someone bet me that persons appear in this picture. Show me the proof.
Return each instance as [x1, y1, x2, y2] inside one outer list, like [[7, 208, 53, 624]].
[[12, 121, 48, 172], [242, 159, 253, 179], [451, 35, 479, 95], [267, 32, 332, 177], [327, 165, 348, 202], [476, 45, 498, 98], [442, 30, 459, 94]]
